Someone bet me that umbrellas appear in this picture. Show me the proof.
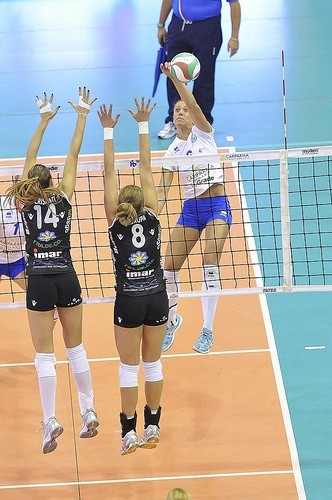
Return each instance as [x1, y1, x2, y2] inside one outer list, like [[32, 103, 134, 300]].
[[152, 43, 167, 97]]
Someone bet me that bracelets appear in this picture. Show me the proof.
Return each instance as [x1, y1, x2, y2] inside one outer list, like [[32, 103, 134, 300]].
[[230, 37, 238, 41], [138, 122, 149, 134], [104, 128, 114, 140], [158, 24, 164, 28]]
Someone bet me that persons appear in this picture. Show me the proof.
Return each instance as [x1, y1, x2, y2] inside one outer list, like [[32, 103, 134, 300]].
[[157, 0, 241, 139], [0, 175, 57, 329], [5, 86, 97, 454], [98, 97, 169, 456], [158, 62, 233, 354]]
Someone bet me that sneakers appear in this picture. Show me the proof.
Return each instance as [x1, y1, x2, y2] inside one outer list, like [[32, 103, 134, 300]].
[[158, 121, 178, 139], [192, 328, 214, 354], [35, 417, 64, 454], [162, 314, 183, 351], [121, 430, 139, 456], [80, 409, 99, 438], [139, 425, 160, 449]]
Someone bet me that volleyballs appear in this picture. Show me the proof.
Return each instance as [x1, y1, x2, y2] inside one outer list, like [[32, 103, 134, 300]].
[[169, 52, 201, 82]]
